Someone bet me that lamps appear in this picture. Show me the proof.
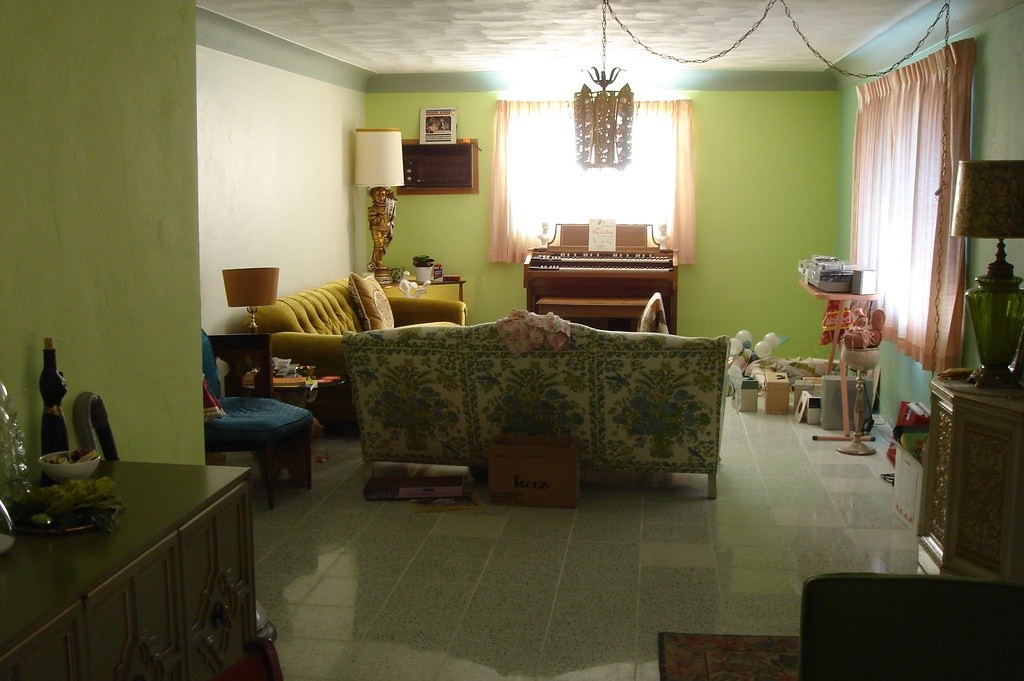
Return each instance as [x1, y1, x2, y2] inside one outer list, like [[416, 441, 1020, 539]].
[[353, 128, 405, 284], [222, 267, 280, 385], [573, 0, 948, 380], [951, 160, 1024, 385]]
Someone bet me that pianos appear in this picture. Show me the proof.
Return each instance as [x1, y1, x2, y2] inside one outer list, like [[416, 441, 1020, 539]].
[[523, 246, 680, 335]]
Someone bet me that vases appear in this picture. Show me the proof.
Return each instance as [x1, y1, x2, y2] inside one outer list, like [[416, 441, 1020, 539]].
[[836, 346, 880, 455]]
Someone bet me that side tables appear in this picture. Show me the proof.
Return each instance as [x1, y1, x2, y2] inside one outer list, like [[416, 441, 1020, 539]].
[[380, 279, 466, 302]]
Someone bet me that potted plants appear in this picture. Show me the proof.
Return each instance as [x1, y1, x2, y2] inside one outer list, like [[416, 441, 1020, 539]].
[[412, 254, 435, 282]]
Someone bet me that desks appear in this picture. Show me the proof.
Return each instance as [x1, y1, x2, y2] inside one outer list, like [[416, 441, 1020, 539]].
[[917, 367, 1024, 583], [242, 369, 347, 409], [799, 279, 876, 441]]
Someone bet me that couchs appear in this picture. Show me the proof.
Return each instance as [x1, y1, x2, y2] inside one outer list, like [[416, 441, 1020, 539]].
[[244, 271, 467, 435], [342, 309, 732, 500]]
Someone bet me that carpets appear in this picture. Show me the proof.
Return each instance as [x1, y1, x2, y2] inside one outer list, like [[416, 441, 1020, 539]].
[[658, 631, 801, 681]]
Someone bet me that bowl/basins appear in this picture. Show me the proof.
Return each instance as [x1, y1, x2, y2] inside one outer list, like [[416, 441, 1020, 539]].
[[40, 451, 100, 481], [297, 366, 315, 375]]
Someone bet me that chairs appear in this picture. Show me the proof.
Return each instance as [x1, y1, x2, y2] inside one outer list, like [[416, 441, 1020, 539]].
[[201, 329, 314, 510], [637, 292, 670, 334]]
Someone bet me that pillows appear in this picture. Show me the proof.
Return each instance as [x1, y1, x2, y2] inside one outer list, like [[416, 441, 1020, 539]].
[[348, 272, 394, 331]]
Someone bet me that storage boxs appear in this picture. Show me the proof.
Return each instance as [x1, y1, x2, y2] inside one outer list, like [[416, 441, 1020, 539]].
[[488, 434, 580, 508], [880, 427, 928, 535], [794, 380, 815, 411], [734, 376, 759, 412], [764, 372, 790, 415]]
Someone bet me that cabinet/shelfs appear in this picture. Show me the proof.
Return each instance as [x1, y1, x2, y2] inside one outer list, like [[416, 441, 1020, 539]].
[[0, 459, 258, 681]]
